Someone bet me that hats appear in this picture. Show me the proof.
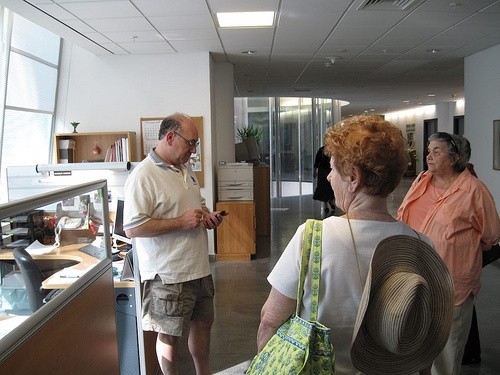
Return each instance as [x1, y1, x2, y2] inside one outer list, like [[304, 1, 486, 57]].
[[348, 235, 453, 372]]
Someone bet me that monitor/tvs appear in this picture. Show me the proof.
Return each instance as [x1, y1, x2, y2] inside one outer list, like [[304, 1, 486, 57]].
[[112, 198, 132, 245]]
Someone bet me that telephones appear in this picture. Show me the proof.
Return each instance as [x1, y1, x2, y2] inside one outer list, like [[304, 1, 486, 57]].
[[55, 216, 82, 229]]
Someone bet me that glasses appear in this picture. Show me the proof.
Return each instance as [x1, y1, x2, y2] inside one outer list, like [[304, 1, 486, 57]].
[[175, 131, 200, 146]]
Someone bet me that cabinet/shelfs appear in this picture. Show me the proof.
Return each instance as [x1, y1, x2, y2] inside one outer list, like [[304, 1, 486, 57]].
[[217, 163, 253, 201], [54, 132, 135, 162], [215, 201, 256, 260], [254, 165, 271, 238]]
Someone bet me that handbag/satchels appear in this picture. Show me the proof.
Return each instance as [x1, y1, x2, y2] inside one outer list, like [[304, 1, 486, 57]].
[[243, 218, 336, 375]]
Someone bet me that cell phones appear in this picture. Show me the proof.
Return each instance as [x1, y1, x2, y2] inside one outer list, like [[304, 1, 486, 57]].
[[206, 210, 225, 225]]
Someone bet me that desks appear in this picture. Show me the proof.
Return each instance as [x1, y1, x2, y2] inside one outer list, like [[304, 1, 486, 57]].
[[0, 240, 140, 375]]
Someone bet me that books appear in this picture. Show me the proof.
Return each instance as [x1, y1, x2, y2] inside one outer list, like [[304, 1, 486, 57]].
[[105, 137, 128, 162]]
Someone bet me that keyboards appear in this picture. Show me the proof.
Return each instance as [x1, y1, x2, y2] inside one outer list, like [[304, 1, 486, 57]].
[[79, 244, 124, 261]]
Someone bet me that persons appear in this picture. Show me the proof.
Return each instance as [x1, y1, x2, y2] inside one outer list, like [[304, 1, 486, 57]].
[[124, 114, 229, 375], [397, 132, 500, 375], [257, 114, 434, 375], [313, 146, 335, 213]]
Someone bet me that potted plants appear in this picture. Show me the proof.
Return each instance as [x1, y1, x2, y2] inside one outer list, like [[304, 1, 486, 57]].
[[405, 149, 417, 177], [234, 123, 264, 166], [71, 122, 80, 133]]
